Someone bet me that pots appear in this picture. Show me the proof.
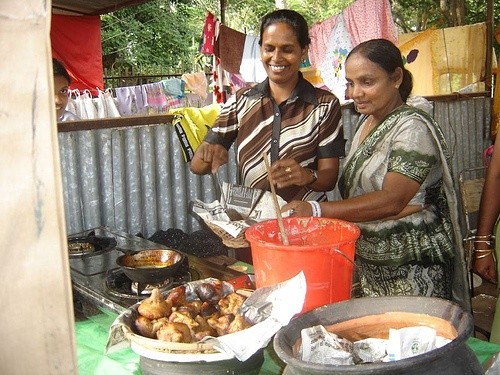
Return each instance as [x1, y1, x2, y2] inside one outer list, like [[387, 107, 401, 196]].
[[113, 247, 183, 284]]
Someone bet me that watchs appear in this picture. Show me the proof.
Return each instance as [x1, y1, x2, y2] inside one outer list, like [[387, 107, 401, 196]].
[[306, 166, 318, 183]]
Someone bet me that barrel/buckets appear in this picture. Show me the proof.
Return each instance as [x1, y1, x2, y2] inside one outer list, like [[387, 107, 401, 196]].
[[245, 216, 361, 317]]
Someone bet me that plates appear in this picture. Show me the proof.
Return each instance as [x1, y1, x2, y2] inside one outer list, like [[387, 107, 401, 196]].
[[468, 272, 482, 289]]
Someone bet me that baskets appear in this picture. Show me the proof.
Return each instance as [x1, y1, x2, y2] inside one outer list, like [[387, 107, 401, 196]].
[[204, 221, 250, 248]]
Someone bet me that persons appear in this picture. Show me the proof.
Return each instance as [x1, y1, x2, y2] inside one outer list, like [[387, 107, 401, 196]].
[[190, 10, 347, 266], [472, 120, 500, 344], [52, 58, 82, 123], [278, 39, 472, 315]]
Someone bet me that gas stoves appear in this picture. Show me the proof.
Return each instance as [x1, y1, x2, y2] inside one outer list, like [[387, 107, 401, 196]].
[[68, 225, 246, 317]]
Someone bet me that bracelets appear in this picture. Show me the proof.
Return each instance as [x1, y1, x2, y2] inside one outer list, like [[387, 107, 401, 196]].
[[463, 234, 497, 270], [306, 201, 321, 218]]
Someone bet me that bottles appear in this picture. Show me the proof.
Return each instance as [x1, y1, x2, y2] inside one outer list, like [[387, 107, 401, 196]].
[[273, 292, 487, 375]]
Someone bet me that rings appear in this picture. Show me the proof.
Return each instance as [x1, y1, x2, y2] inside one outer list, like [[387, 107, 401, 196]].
[[288, 173, 292, 179], [284, 167, 292, 174], [288, 208, 295, 215]]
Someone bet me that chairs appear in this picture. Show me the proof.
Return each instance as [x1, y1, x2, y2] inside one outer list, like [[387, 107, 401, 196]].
[[459, 166, 489, 294]]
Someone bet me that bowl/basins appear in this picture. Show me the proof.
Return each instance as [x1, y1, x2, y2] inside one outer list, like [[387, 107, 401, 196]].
[[123, 305, 264, 375]]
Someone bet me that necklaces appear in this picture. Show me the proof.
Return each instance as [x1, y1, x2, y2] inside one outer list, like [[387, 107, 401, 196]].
[[367, 117, 374, 133]]
